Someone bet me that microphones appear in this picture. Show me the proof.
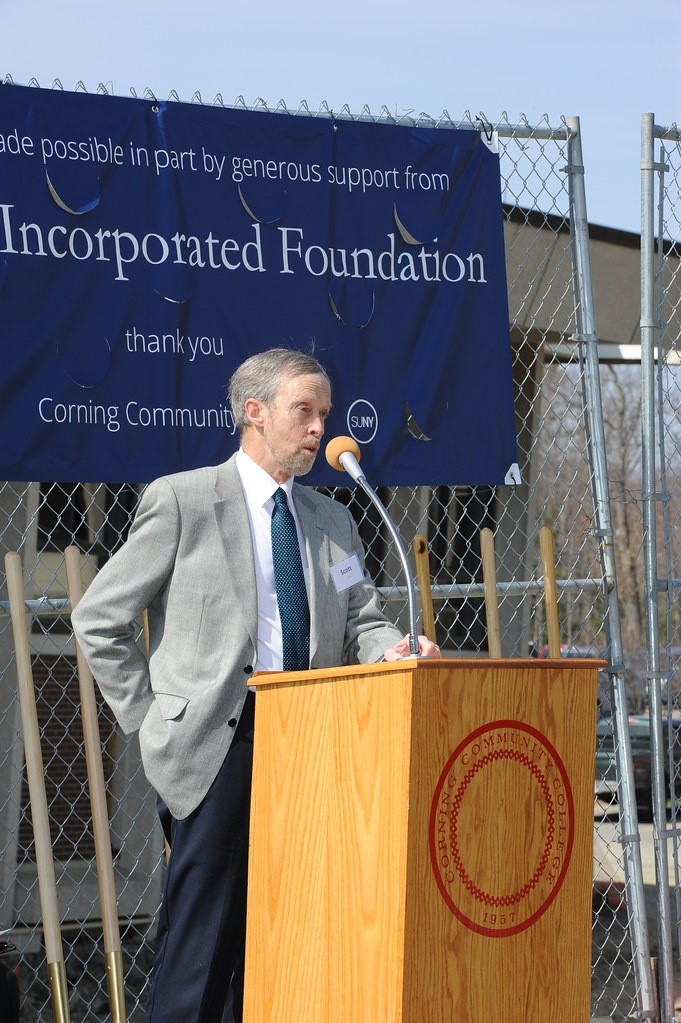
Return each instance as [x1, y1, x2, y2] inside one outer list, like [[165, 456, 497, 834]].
[[325, 434, 419, 656]]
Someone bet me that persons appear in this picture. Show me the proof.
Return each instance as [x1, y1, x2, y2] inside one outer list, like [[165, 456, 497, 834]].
[[69, 346, 441, 1023]]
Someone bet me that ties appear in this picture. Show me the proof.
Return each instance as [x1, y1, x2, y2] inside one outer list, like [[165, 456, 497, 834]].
[[271, 488, 310, 671]]
